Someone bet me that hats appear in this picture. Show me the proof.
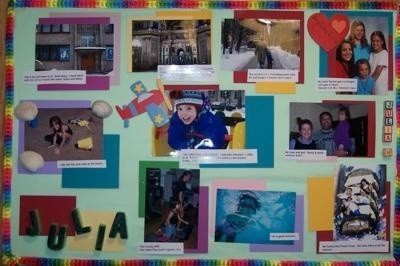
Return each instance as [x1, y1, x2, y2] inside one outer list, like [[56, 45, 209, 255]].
[[176, 90, 204, 106]]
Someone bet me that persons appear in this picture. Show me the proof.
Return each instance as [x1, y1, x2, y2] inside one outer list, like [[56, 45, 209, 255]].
[[266, 48, 274, 68], [47, 116, 74, 155], [342, 197, 353, 216], [254, 37, 266, 67], [333, 108, 352, 151], [215, 192, 261, 242], [370, 31, 389, 95], [328, 39, 354, 78], [176, 171, 193, 229], [289, 118, 317, 149], [154, 200, 181, 240], [168, 90, 228, 149], [343, 189, 360, 203], [350, 60, 375, 94], [348, 20, 372, 64], [361, 179, 372, 194], [313, 112, 349, 156]]
